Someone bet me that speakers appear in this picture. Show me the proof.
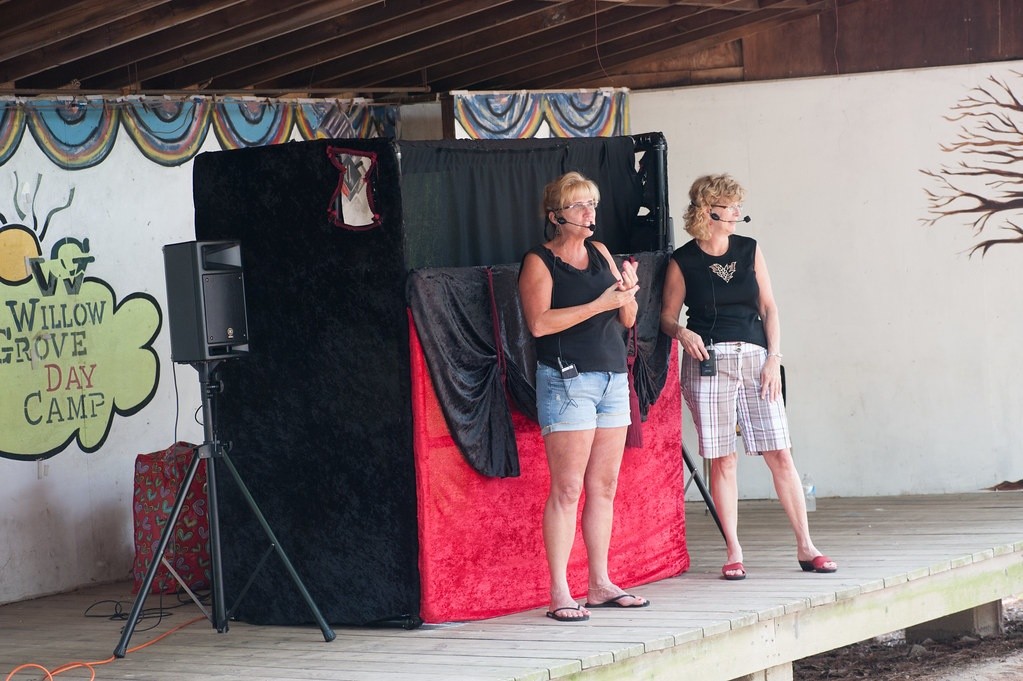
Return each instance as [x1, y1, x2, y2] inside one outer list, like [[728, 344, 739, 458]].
[[163, 240, 251, 365]]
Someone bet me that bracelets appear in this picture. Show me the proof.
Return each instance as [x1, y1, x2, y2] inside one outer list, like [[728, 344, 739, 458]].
[[767, 353, 782, 358]]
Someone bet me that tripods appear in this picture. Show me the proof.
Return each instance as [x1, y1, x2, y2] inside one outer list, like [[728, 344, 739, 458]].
[[114, 360, 336, 660]]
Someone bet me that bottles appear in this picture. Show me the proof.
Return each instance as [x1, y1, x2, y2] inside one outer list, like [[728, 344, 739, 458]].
[[801, 473, 816, 512]]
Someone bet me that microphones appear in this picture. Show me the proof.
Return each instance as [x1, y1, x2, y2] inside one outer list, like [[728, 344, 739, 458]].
[[555, 216, 596, 231], [710, 213, 751, 223]]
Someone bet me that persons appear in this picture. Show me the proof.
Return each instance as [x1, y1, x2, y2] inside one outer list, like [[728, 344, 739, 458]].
[[520, 172, 650, 621], [661, 173, 837, 580]]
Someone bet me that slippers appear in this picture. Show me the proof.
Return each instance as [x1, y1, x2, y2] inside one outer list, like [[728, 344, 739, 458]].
[[585, 594, 649, 608], [547, 605, 589, 621]]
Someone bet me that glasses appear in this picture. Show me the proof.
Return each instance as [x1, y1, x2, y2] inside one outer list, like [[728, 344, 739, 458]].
[[711, 203, 742, 211], [555, 200, 598, 211]]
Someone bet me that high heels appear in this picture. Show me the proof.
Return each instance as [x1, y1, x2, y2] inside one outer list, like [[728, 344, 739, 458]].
[[799, 555, 836, 573], [723, 561, 745, 580]]
